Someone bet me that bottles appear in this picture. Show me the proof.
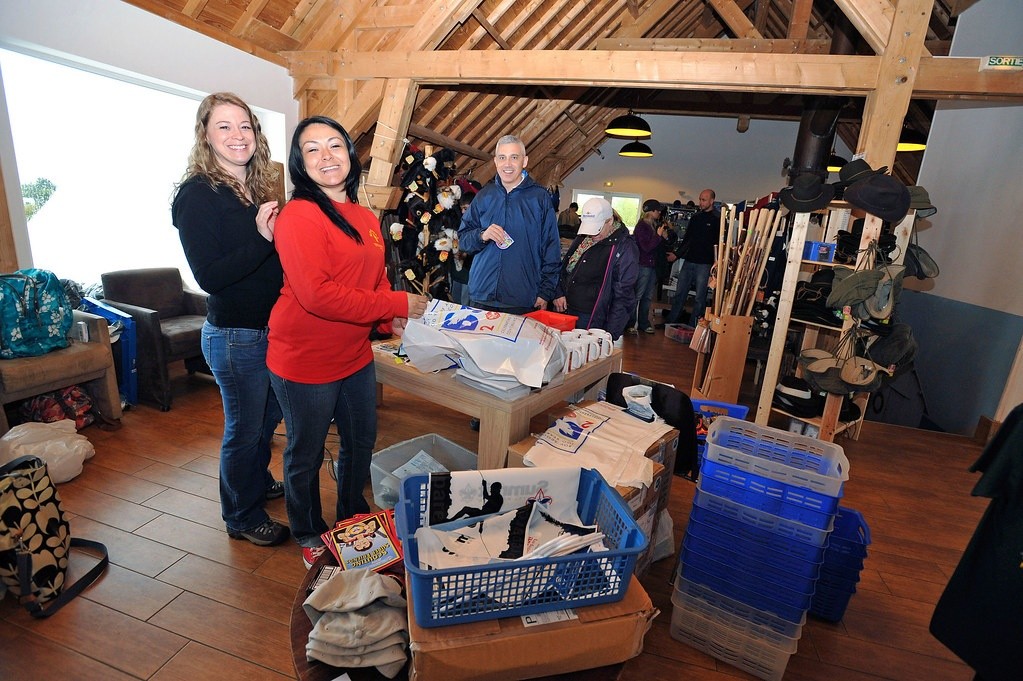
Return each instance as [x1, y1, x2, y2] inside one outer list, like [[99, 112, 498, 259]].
[[562, 210, 576, 226]]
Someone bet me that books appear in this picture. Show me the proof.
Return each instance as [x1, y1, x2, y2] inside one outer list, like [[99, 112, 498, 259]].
[[321, 509, 404, 574]]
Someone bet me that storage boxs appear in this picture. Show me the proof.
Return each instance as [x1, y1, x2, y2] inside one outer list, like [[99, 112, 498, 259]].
[[691, 307, 754, 404], [647, 302, 672, 325], [369, 432, 479, 511], [669, 399, 872, 681], [802, 241, 838, 264], [393, 465, 651, 630], [505, 428, 666, 575], [546, 401, 680, 514], [664, 323, 696, 344], [521, 310, 580, 333], [403, 565, 661, 681]]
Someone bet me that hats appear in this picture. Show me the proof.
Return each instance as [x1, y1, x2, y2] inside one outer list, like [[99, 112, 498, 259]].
[[772, 244, 939, 422], [577, 198, 613, 235], [781, 159, 937, 222], [643, 200, 667, 212]]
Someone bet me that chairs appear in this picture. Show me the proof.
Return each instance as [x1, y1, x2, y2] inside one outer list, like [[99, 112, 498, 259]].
[[98, 268, 214, 412], [0, 307, 125, 437]]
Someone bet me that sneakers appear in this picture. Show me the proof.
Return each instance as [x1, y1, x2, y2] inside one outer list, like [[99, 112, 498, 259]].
[[268, 481, 284, 498], [226, 519, 289, 545]]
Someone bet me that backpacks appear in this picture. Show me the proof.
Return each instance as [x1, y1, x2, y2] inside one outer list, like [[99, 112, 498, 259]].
[[22, 385, 99, 431]]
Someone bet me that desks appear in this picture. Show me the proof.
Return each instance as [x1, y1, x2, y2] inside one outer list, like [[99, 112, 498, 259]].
[[288, 549, 410, 681], [371, 338, 623, 470]]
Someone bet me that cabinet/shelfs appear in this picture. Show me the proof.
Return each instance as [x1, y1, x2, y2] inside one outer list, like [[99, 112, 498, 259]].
[[754, 200, 917, 441], [663, 204, 700, 246]]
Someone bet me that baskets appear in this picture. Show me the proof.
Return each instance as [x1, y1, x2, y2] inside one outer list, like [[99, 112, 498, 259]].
[[670, 399, 869, 681], [394, 467, 648, 627], [665, 323, 696, 343]]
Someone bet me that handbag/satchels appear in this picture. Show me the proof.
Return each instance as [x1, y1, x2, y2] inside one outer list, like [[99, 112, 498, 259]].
[[0, 418, 95, 484], [0, 268, 73, 359], [400, 297, 567, 387], [0, 455, 108, 617]]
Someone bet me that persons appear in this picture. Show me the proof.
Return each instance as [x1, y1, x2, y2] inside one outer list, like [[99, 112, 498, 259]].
[[625, 199, 677, 335], [655, 189, 728, 329], [558, 201, 581, 227], [552, 198, 639, 341], [264, 116, 428, 571], [457, 135, 560, 315], [172, 92, 289, 548], [450, 191, 476, 305]]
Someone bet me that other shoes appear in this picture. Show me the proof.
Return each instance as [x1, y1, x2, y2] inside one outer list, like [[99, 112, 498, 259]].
[[303, 545, 327, 570], [627, 328, 637, 334], [644, 327, 655, 333]]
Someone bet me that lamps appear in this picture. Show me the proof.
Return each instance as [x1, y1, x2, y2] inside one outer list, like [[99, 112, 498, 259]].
[[826, 122, 849, 173], [618, 139, 653, 158], [896, 114, 928, 152], [604, 107, 652, 137]]
